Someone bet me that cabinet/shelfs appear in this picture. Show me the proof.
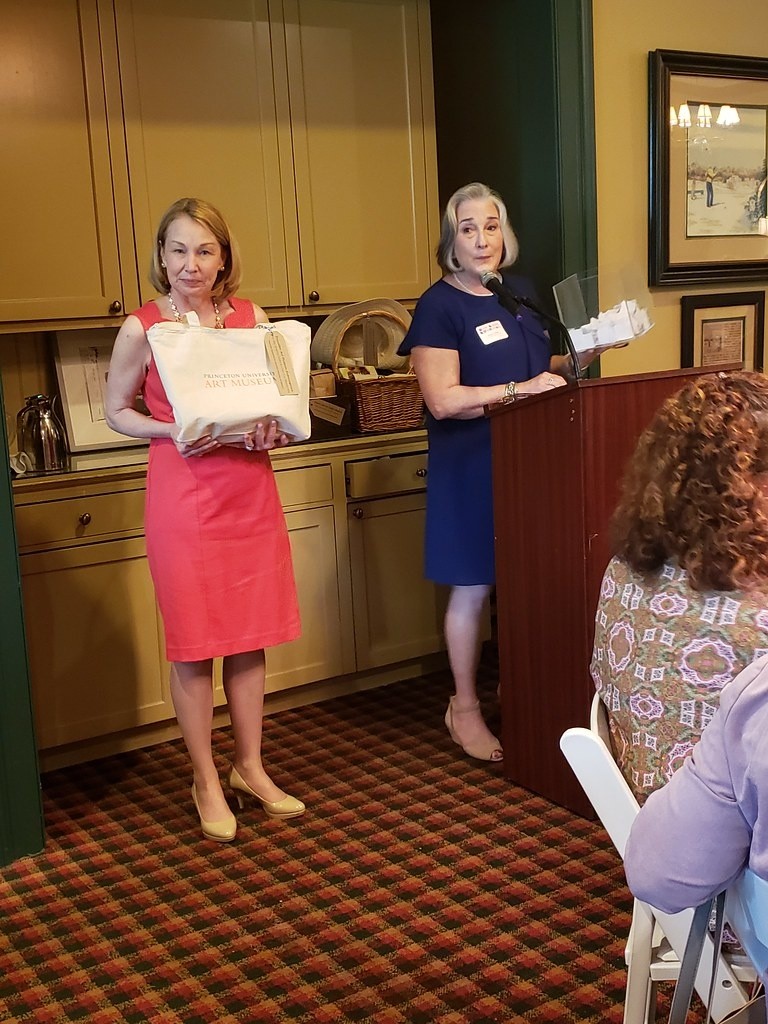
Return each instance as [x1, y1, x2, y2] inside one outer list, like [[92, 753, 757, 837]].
[[10, 435, 490, 774], [0, 0, 443, 330]]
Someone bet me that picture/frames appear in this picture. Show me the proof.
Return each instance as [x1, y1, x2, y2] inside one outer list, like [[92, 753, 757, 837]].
[[647, 49, 768, 287], [683, 290, 764, 376], [48, 330, 152, 453]]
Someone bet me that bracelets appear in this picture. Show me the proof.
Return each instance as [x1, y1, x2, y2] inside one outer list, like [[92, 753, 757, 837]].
[[505, 381, 516, 396]]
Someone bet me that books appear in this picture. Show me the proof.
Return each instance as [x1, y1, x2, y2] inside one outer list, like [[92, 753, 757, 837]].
[[339, 366, 379, 380]]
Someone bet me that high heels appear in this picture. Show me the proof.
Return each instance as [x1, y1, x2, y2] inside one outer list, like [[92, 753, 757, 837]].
[[191, 780, 238, 842], [228, 764, 306, 819], [446, 683, 507, 762]]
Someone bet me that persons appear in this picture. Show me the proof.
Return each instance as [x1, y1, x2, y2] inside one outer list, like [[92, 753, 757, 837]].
[[587, 369, 768, 993], [395, 184, 628, 760], [348, 366, 370, 379], [103, 197, 306, 840]]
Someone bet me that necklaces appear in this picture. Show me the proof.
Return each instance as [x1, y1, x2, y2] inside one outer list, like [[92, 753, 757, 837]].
[[451, 270, 493, 296], [168, 291, 223, 329]]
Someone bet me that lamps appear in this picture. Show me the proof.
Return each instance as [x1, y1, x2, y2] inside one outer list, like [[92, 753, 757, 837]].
[[667, 102, 741, 127]]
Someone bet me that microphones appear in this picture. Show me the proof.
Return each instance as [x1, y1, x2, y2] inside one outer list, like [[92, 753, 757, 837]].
[[479, 270, 523, 321]]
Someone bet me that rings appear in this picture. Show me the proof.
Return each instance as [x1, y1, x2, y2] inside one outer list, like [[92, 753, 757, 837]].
[[244, 444, 255, 450], [548, 377, 554, 383]]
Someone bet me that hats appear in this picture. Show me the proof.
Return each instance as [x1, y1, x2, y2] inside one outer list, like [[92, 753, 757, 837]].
[[310, 296, 414, 368]]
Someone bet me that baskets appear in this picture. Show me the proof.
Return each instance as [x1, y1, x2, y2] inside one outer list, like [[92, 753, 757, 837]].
[[330, 312, 426, 434]]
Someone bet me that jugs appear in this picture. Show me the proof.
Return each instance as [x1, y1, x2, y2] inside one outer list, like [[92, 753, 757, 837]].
[[16, 393, 71, 472]]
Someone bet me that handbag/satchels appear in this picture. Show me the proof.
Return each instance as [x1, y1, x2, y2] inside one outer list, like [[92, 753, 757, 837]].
[[147, 312, 312, 442]]
